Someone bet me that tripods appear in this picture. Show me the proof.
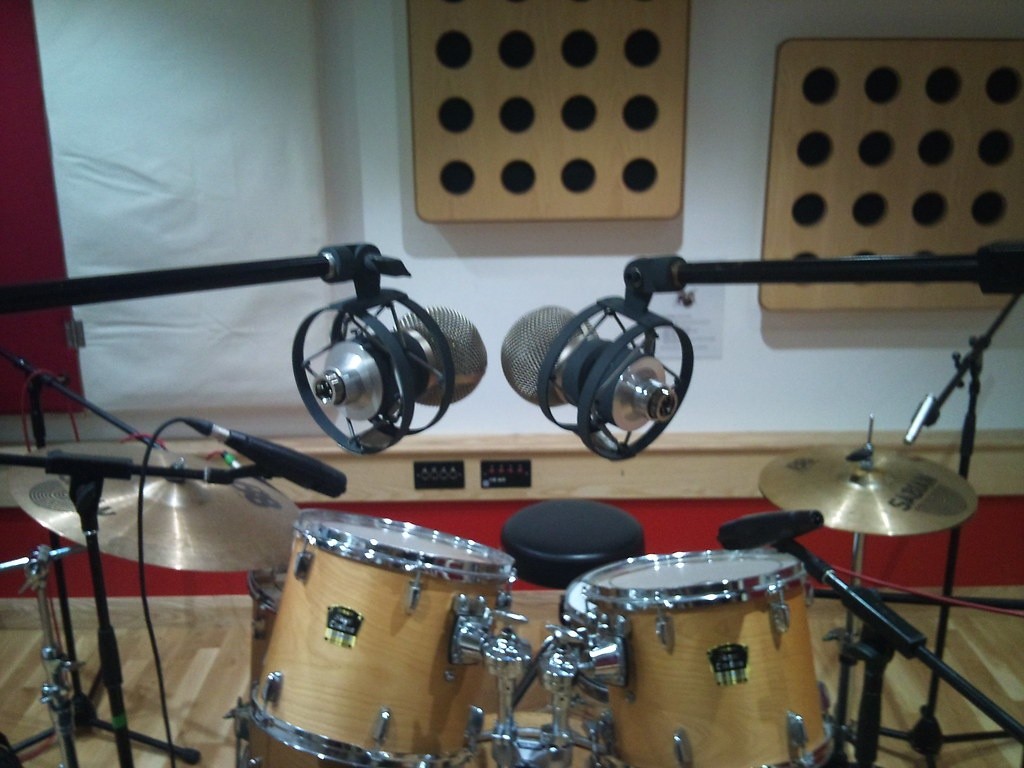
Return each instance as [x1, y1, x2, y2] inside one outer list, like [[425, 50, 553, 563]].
[[0, 526, 202, 767], [880, 294, 1021, 767]]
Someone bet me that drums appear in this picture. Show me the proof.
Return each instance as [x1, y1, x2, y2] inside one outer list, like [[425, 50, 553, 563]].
[[556, 548, 832, 768], [240, 498, 522, 768]]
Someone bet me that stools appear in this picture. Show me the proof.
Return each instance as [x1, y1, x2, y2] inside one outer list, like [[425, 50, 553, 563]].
[[501, 498, 644, 768]]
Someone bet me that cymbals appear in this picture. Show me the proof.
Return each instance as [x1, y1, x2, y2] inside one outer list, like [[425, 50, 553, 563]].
[[757, 440, 979, 539], [6, 444, 301, 578]]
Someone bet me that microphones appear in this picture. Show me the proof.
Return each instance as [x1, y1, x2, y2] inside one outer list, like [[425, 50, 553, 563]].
[[715, 508, 820, 551], [184, 409, 349, 497], [294, 293, 488, 457], [501, 293, 696, 463]]
